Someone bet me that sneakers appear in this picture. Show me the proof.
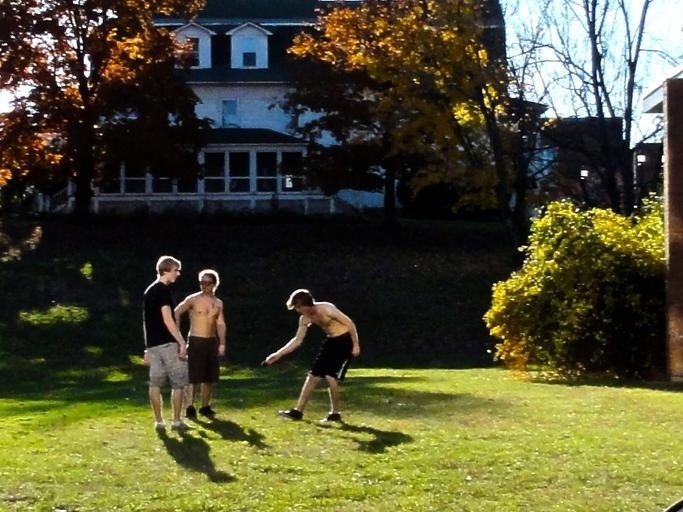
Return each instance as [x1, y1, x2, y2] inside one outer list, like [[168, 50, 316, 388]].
[[185, 406, 196, 417], [171, 422, 195, 431], [199, 404, 216, 415], [156, 423, 166, 432], [278, 407, 303, 420], [321, 413, 341, 422]]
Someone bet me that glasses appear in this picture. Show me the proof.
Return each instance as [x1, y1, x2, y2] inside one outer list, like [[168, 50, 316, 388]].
[[199, 280, 216, 285], [175, 269, 183, 273]]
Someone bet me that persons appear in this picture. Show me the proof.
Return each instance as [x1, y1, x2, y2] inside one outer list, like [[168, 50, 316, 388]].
[[260, 289, 359, 423], [141, 256, 196, 431], [174, 269, 227, 420]]
